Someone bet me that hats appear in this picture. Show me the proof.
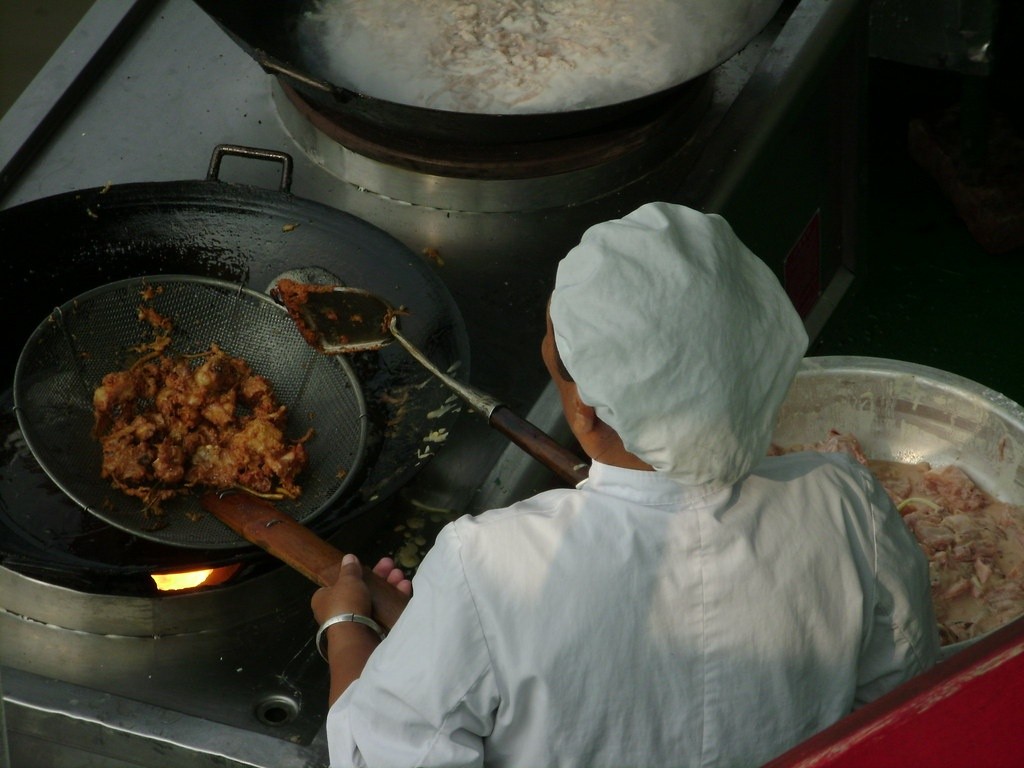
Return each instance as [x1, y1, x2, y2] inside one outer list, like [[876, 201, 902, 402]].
[[549, 202, 809, 487]]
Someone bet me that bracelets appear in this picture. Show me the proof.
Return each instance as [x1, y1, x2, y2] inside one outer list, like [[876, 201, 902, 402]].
[[315, 611, 387, 663]]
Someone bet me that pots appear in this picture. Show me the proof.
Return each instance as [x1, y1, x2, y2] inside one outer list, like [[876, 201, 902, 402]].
[[0, 144, 479, 587], [185, 1, 796, 155]]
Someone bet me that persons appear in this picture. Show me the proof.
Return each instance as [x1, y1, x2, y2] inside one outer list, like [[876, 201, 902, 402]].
[[310, 203, 941, 767]]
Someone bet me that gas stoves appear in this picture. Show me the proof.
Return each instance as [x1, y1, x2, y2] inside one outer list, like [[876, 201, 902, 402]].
[[1, 0, 886, 768]]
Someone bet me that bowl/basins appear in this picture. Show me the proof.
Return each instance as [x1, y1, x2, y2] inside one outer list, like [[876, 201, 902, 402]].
[[747, 353, 1024, 667]]
